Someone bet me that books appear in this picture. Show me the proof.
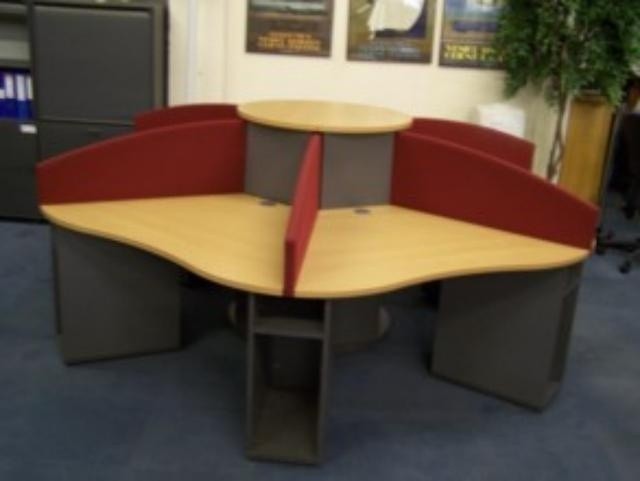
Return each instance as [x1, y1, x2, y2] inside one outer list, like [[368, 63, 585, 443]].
[[0, 71, 33, 120]]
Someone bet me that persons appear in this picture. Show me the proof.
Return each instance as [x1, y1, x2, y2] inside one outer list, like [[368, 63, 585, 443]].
[[610, 62, 640, 218]]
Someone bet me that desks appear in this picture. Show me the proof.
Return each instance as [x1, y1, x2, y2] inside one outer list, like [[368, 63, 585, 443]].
[[26, 100, 604, 466]]
